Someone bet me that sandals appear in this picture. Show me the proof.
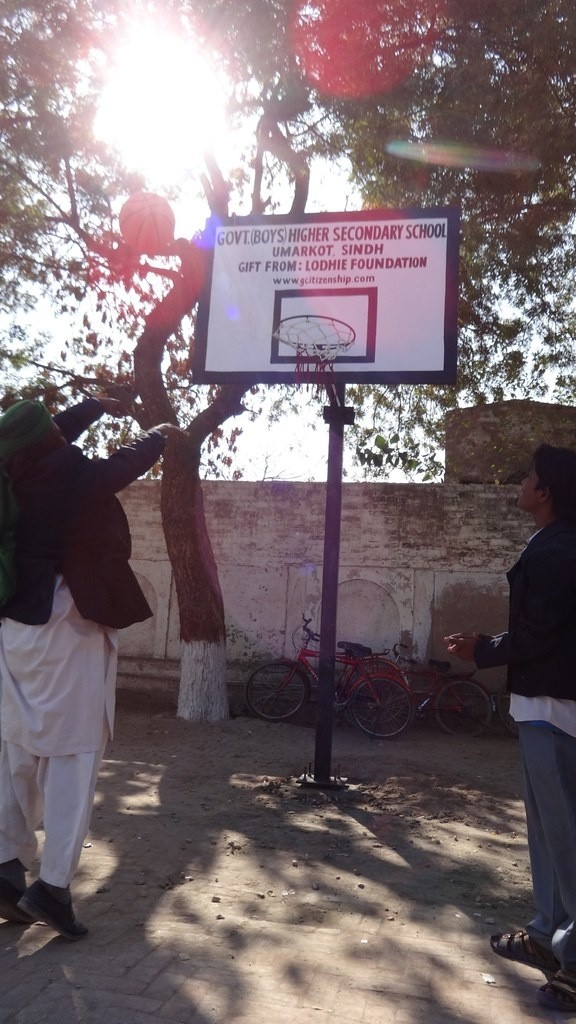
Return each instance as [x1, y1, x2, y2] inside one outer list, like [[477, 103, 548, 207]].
[[490, 930, 560, 970], [537, 969, 576, 1011]]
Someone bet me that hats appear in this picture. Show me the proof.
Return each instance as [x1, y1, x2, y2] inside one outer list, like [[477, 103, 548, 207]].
[[0, 399, 51, 454]]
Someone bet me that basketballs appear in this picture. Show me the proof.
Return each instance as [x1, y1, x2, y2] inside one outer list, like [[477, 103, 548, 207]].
[[119, 192, 175, 251]]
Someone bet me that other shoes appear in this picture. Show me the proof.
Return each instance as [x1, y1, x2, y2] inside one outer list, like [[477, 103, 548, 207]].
[[17, 881, 88, 940], [0, 876, 38, 923]]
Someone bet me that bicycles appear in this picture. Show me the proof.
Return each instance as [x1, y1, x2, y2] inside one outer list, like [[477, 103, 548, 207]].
[[349, 642, 497, 741], [244, 611, 418, 743]]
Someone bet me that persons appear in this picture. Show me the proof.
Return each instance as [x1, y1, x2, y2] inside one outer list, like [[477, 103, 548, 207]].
[[444, 441, 576, 1011], [1, 396, 191, 943]]
